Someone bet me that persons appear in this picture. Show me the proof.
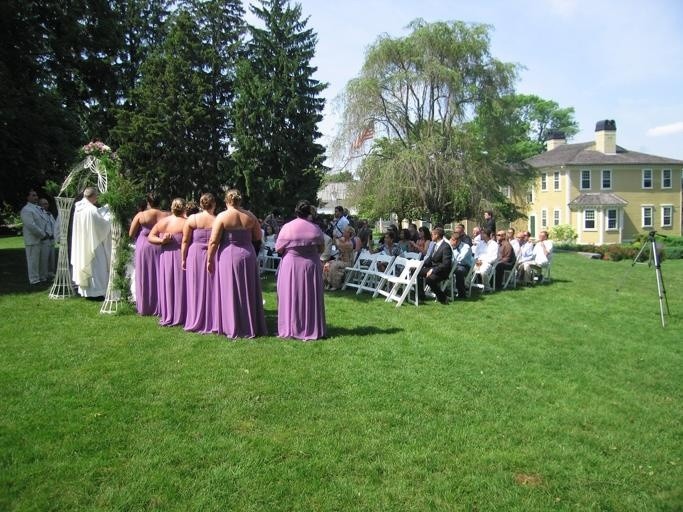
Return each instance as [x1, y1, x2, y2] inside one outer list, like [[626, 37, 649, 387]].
[[207, 189, 263, 338], [455, 224, 473, 248], [495, 231, 516, 289], [20, 190, 49, 288], [147, 198, 189, 323], [410, 226, 432, 256], [38, 199, 57, 278], [128, 190, 166, 316], [516, 231, 554, 286], [482, 210, 496, 239], [379, 224, 399, 243], [398, 229, 410, 253], [473, 229, 499, 293], [412, 228, 452, 305], [371, 231, 401, 274], [507, 228, 520, 258], [448, 232, 472, 298], [240, 195, 263, 256], [310, 204, 325, 230], [520, 231, 534, 264], [322, 226, 357, 291], [181, 193, 216, 333], [71, 187, 114, 298], [408, 224, 419, 242], [265, 225, 278, 257], [276, 200, 326, 341], [332, 206, 350, 238]]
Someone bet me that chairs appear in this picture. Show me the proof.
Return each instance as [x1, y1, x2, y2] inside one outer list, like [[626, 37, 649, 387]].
[[423, 253, 457, 303], [465, 256, 496, 297], [501, 256, 520, 289], [403, 251, 422, 260], [341, 250, 380, 295], [372, 256, 409, 302], [530, 262, 551, 284], [388, 259, 425, 308], [356, 254, 395, 299]]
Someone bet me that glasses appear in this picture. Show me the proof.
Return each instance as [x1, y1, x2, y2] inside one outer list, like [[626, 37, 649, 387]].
[[495, 234, 500, 236]]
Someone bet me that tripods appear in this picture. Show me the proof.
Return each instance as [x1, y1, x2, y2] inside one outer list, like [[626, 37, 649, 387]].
[[616, 229, 671, 327]]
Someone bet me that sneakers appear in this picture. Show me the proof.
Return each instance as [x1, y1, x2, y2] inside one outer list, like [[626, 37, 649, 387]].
[[330, 287, 338, 291], [86, 296, 105, 301]]
